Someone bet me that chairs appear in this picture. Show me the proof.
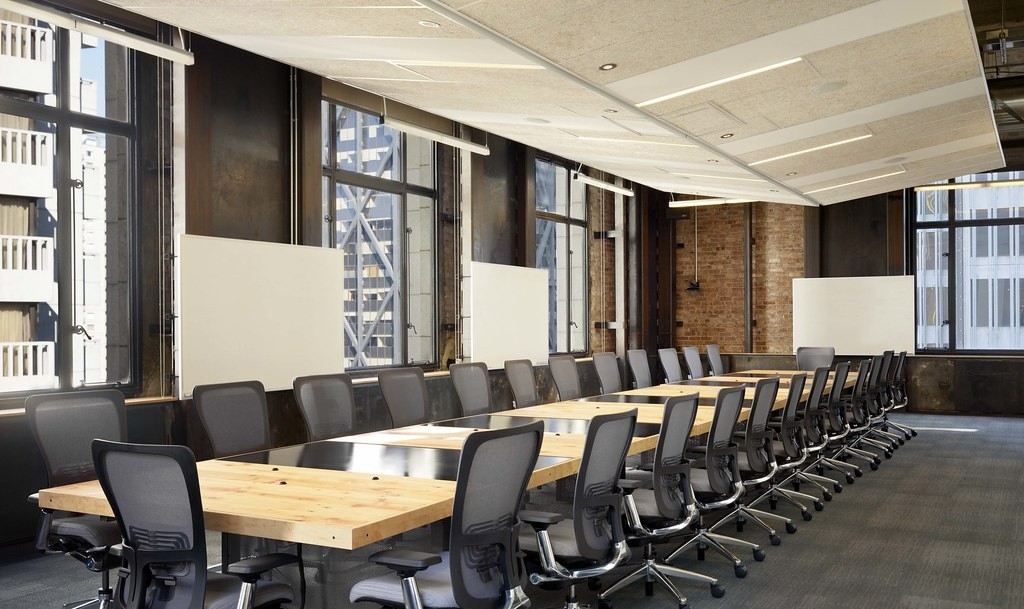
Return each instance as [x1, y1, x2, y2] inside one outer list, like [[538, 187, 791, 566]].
[[597, 392, 726, 609], [793, 350, 917, 485], [374, 367, 430, 428], [192, 379, 327, 583], [774, 368, 842, 503], [349, 420, 544, 609], [91, 439, 307, 609], [504, 345, 725, 408], [25, 388, 140, 609], [705, 376, 797, 547], [660, 383, 766, 578], [294, 374, 404, 550], [449, 362, 493, 416], [515, 407, 638, 609], [745, 372, 825, 521], [797, 346, 835, 371]]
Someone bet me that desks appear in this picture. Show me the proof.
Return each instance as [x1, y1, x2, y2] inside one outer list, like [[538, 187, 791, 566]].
[[38, 367, 870, 609]]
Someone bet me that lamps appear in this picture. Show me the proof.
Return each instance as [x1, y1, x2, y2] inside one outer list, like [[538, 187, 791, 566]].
[[914, 179, 1024, 192], [380, 115, 490, 157], [0, 0, 195, 66], [669, 198, 758, 207], [574, 173, 634, 196]]
